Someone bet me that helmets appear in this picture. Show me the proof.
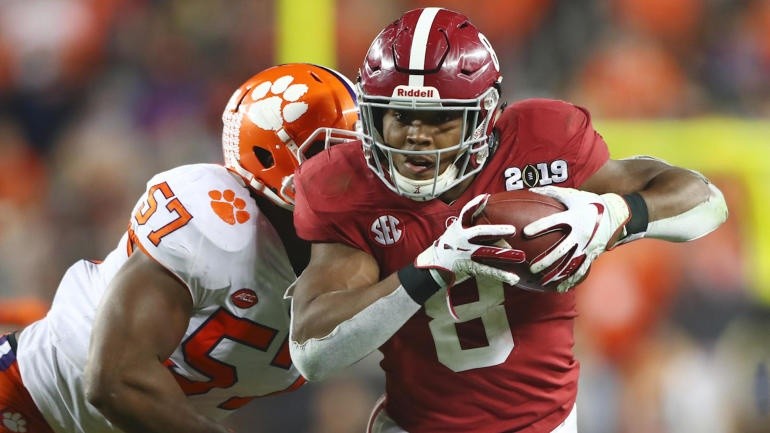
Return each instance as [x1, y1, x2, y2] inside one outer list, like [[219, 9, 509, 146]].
[[355, 5, 505, 201], [220, 61, 370, 213]]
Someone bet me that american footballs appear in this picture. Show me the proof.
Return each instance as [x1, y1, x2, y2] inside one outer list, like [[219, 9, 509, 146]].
[[472, 190, 592, 289]]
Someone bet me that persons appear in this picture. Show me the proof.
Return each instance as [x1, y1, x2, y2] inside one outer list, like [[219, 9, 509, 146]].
[[0, 63, 370, 433], [283, 6, 730, 433]]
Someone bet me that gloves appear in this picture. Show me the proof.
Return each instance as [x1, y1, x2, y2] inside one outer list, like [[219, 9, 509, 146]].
[[414, 192, 527, 324], [523, 184, 649, 296]]
